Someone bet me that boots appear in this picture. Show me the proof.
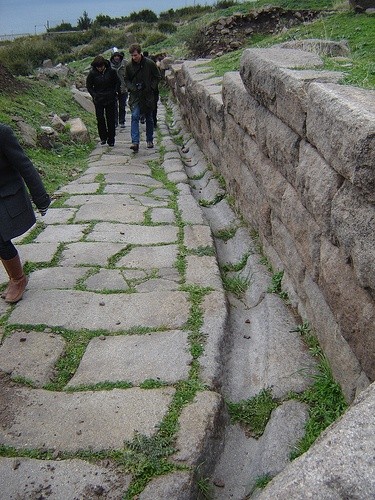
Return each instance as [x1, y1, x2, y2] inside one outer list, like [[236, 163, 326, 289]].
[[2, 254, 28, 302], [1, 259, 12, 299]]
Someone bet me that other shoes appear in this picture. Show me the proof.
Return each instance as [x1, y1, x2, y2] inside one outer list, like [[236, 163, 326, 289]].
[[147, 141, 153, 148], [100, 138, 107, 145], [130, 144, 139, 152], [107, 138, 115, 146]]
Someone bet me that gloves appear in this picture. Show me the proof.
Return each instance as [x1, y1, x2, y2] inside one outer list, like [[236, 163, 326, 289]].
[[38, 206, 48, 216]]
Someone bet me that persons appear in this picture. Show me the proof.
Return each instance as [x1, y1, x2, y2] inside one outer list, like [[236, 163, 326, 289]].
[[0, 123, 51, 302], [123, 44, 161, 153], [143, 50, 148, 58], [149, 56, 160, 128], [86, 56, 122, 147], [110, 51, 128, 129]]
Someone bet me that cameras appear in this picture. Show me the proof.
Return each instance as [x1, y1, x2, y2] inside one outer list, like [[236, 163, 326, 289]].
[[136, 83, 144, 91]]
